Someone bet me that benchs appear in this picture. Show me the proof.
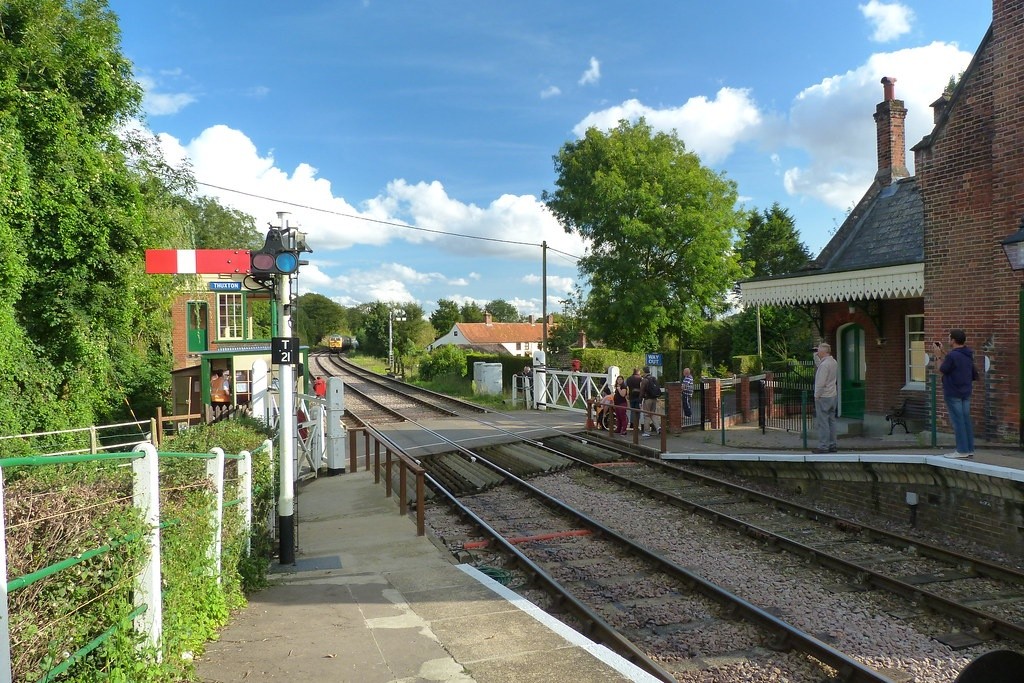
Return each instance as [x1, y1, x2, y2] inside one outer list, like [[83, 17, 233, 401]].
[[886, 398, 909, 436]]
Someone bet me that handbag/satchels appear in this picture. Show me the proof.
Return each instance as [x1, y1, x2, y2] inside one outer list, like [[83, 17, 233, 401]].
[[971, 364, 980, 381]]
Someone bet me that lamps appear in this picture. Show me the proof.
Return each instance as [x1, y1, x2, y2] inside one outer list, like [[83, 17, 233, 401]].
[[849, 301, 856, 313]]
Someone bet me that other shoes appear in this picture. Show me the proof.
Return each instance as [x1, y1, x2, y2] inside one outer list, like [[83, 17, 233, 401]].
[[656, 427, 661, 435], [684, 415, 693, 420], [812, 448, 830, 454], [641, 433, 651, 437], [620, 432, 628, 436], [944, 450, 974, 458], [830, 446, 837, 452]]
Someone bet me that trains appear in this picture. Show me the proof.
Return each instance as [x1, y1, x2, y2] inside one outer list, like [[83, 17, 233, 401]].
[[327, 334, 360, 354]]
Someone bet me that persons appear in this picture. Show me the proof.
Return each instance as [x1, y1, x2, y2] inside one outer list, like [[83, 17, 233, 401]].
[[933, 331, 975, 458], [638, 366, 661, 437], [210, 369, 232, 411], [626, 368, 642, 428], [812, 343, 838, 454], [600, 387, 611, 407], [614, 376, 628, 435], [313, 376, 326, 397], [681, 368, 693, 419], [523, 366, 533, 398]]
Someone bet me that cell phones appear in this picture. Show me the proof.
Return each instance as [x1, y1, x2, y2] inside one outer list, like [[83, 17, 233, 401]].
[[935, 342, 940, 346]]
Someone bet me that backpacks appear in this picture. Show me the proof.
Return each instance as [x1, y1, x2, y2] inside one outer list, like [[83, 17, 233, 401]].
[[645, 378, 662, 398]]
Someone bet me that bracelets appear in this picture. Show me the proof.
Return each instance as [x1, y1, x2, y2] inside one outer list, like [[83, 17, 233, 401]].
[[936, 357, 941, 360]]
